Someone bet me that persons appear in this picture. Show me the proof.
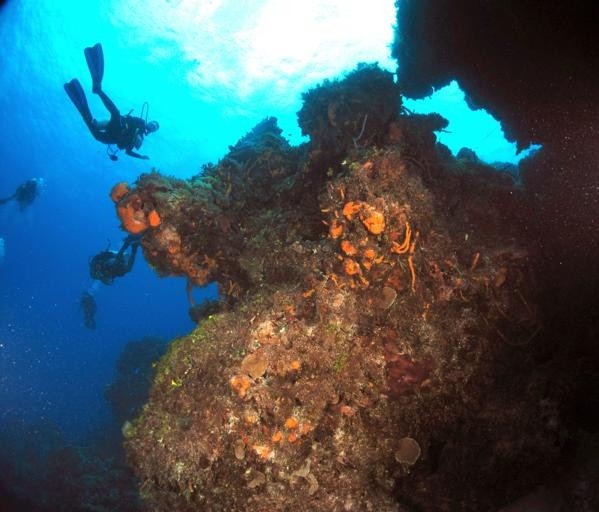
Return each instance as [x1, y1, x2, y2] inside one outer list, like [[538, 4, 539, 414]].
[[0, 179, 39, 213], [79, 292, 97, 330], [62, 42, 159, 161], [90, 233, 140, 286]]
